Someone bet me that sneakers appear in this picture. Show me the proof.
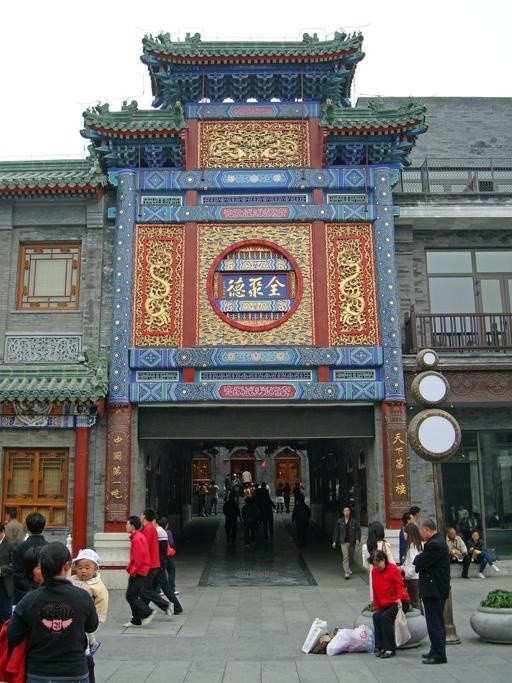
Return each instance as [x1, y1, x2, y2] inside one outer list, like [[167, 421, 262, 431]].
[[123, 602, 183, 627], [477, 571, 485, 578], [462, 573, 470, 579], [89, 640, 101, 656], [349, 569, 352, 574], [492, 564, 499, 572], [344, 572, 349, 578]]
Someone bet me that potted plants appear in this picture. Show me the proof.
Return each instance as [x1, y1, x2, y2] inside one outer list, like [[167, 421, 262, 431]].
[[356, 601, 427, 651], [467, 589, 512, 645]]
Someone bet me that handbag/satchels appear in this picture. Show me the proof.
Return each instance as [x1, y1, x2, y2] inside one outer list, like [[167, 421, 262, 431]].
[[449, 549, 461, 557], [300, 617, 340, 654], [166, 531, 176, 558], [394, 608, 412, 647]]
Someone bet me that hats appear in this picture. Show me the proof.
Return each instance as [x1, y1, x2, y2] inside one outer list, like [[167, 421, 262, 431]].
[[71, 549, 103, 569]]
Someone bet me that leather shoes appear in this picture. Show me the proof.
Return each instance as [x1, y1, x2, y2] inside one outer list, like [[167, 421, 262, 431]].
[[376, 649, 396, 658], [422, 652, 435, 658], [422, 657, 446, 664]]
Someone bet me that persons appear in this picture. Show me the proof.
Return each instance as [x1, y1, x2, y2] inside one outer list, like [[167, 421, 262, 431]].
[[151, 513, 183, 615], [131, 509, 174, 619], [332, 506, 360, 578], [65, 549, 109, 657], [413, 518, 450, 663], [362, 506, 500, 607], [7, 541, 98, 683], [23, 545, 95, 683], [198, 468, 311, 547], [123, 516, 156, 627], [157, 517, 179, 596], [369, 550, 410, 658], [0, 509, 50, 625]]
